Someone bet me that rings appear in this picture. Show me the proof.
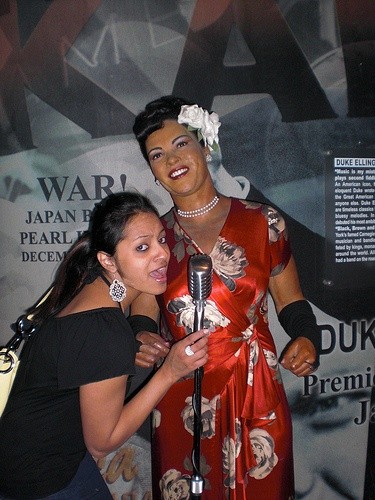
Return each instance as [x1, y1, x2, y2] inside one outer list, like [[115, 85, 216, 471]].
[[185, 344, 195, 357], [304, 361, 313, 368]]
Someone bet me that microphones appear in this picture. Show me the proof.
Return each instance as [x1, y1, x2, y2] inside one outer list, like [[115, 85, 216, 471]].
[[188, 254, 212, 332]]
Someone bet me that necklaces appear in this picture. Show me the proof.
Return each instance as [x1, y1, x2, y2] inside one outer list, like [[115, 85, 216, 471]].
[[99, 270, 122, 309], [177, 195, 220, 217]]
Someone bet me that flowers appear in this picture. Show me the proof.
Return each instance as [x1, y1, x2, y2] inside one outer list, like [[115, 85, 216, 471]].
[[177, 104, 206, 133], [198, 107, 222, 153]]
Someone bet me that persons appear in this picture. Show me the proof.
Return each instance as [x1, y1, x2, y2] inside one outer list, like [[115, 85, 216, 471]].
[[128, 96, 322, 500], [0, 193, 209, 500]]
